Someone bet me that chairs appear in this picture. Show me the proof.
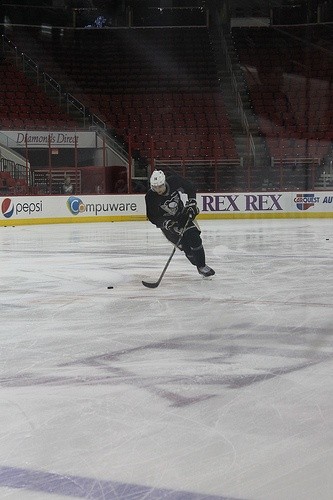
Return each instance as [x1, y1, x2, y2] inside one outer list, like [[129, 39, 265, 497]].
[[0, 16, 332, 197]]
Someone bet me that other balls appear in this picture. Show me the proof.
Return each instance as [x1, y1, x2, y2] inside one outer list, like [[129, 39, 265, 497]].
[[107, 287, 113, 289], [326, 239, 329, 240]]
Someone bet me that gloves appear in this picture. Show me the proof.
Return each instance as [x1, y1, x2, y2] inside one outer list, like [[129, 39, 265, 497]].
[[164, 220, 183, 237], [186, 202, 197, 221]]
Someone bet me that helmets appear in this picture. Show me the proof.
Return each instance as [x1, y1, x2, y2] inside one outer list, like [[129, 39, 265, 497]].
[[150, 170, 165, 187]]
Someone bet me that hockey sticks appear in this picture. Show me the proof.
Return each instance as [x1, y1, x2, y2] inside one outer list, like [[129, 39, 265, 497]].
[[142, 210, 192, 288]]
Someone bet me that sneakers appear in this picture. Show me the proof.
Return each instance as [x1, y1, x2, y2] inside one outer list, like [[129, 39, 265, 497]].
[[197, 267, 211, 280], [204, 264, 216, 280]]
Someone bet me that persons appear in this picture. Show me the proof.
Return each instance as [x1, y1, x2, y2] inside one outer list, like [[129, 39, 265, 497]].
[[71, 184, 76, 195], [61, 176, 73, 195], [145, 169, 216, 277]]
